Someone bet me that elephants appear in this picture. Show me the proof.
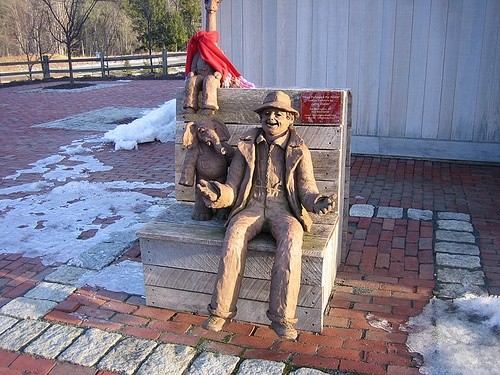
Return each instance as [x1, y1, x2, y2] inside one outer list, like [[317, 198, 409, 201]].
[[178, 117, 236, 225]]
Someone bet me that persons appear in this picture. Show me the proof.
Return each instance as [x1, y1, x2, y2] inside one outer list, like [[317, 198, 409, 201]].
[[189, 89, 340, 340]]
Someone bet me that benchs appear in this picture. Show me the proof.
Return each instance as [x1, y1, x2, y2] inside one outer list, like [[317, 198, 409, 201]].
[[134, 86, 353, 334]]
